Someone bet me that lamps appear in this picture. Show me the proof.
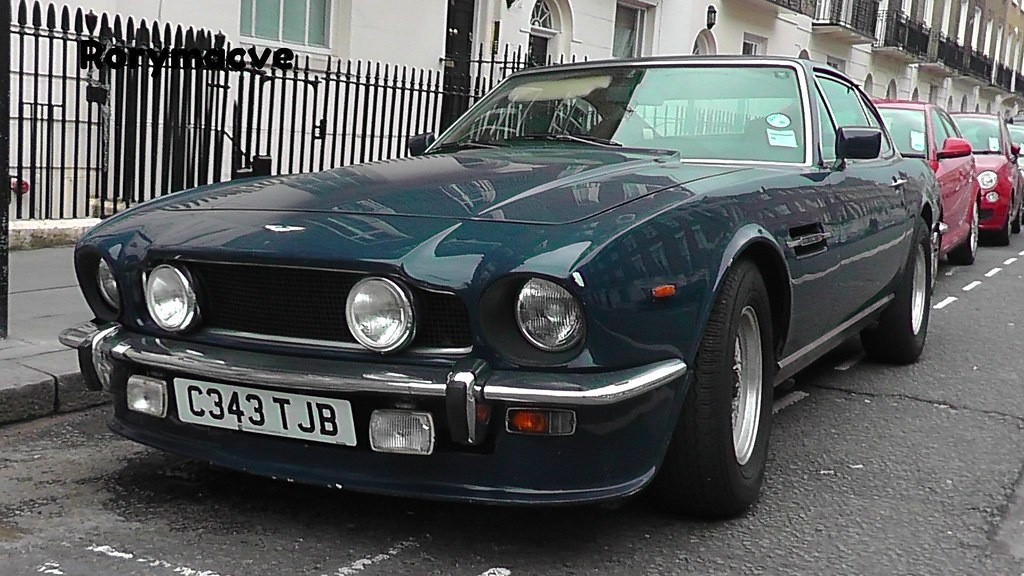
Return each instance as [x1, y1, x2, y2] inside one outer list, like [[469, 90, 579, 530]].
[[707, 5, 717, 29]]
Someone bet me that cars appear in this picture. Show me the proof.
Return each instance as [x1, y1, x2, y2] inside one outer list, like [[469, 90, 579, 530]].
[[60, 55, 944, 512], [744, 100, 981, 265], [947, 112, 1024, 244]]
